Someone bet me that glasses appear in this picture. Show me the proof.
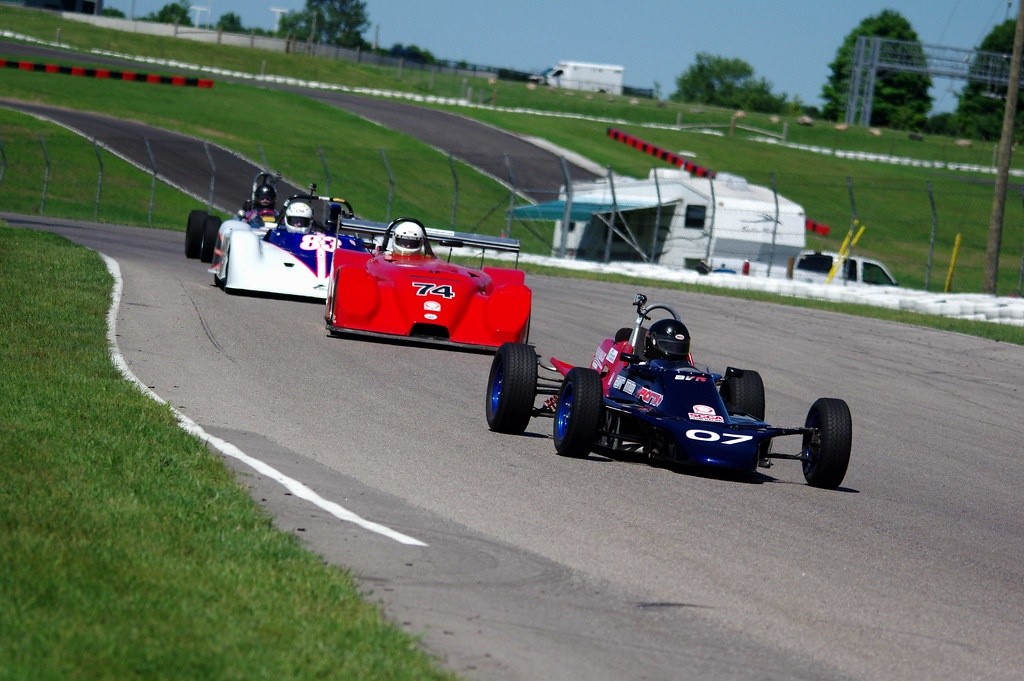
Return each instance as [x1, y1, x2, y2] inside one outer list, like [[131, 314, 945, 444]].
[[292, 219, 307, 225], [257, 195, 272, 201]]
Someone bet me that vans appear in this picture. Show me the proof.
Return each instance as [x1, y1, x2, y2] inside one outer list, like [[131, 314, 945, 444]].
[[793, 248, 898, 286]]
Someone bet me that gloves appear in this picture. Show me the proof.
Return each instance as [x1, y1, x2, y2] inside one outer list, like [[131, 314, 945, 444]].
[[707, 368, 722, 384]]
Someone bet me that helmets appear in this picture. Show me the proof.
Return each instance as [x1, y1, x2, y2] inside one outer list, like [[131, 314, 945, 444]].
[[285, 202, 313, 233], [644, 319, 691, 361], [254, 184, 276, 209], [392, 221, 424, 255]]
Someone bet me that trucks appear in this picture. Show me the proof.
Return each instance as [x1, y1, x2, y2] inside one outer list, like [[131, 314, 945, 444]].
[[534, 62, 626, 97]]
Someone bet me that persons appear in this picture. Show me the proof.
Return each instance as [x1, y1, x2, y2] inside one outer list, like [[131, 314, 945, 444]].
[[284, 202, 313, 234], [392, 222, 423, 254], [254, 184, 276, 208], [642, 319, 689, 363]]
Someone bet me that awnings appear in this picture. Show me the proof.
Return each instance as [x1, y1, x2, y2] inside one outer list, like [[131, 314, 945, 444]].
[[506, 199, 684, 263]]
